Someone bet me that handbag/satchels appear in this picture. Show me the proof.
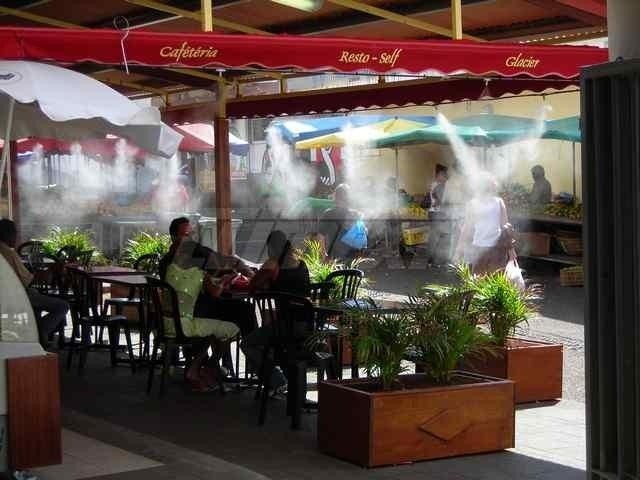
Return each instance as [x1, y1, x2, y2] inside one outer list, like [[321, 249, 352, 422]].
[[341, 220, 368, 250], [497, 223, 520, 248]]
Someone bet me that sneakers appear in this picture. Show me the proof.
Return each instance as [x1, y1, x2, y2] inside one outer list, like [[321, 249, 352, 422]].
[[263, 369, 288, 400]]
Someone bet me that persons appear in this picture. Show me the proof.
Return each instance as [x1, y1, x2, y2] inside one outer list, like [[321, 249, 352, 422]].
[[158, 216, 222, 282], [316, 184, 368, 262], [249, 230, 315, 396], [0, 248, 43, 480], [522, 162, 552, 205], [0, 219, 71, 347], [453, 173, 526, 299], [156, 240, 241, 393], [424, 160, 448, 274]]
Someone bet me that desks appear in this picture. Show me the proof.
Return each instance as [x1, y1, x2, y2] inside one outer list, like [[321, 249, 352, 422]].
[[509, 212, 587, 270]]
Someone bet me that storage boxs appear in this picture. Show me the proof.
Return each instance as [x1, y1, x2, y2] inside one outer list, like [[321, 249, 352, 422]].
[[518, 231, 552, 257]]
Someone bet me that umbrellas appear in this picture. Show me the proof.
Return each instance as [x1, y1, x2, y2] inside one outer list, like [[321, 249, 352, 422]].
[[1, 59, 184, 187]]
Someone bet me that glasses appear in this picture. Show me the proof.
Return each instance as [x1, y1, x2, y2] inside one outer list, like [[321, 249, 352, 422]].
[[176, 231, 190, 236]]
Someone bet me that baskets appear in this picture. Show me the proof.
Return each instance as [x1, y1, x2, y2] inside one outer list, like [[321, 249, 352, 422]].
[[559, 267, 584, 287], [403, 229, 429, 245]]
